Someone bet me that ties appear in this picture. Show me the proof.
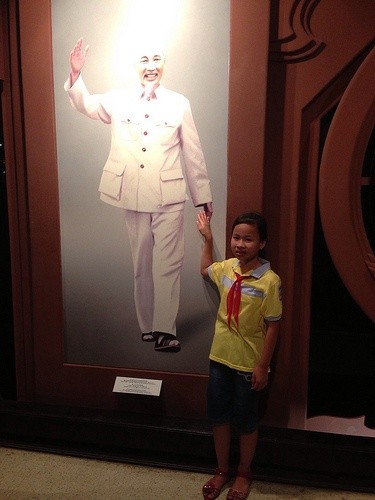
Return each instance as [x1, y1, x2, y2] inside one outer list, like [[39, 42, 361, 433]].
[[225, 272, 252, 330]]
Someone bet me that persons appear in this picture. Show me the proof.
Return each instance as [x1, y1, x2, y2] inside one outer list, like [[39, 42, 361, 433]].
[[63, 36, 214, 352], [196, 210, 283, 500]]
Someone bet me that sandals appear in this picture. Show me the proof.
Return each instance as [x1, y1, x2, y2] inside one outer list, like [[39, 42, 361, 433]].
[[203, 470, 231, 500], [227, 474, 252, 500], [142, 332, 155, 342], [154, 334, 181, 352]]
